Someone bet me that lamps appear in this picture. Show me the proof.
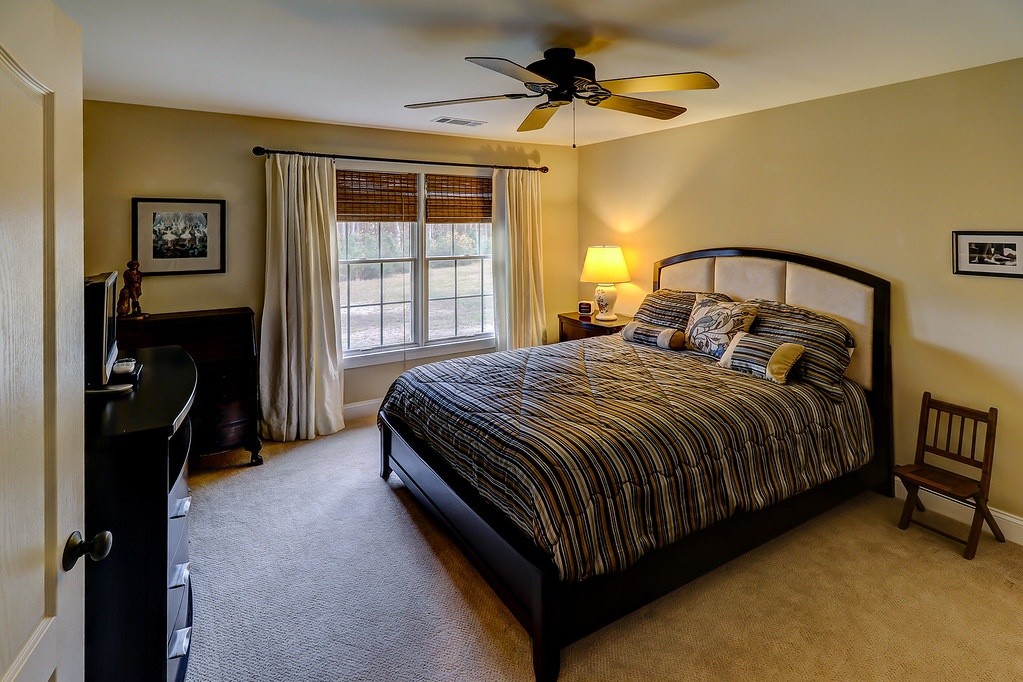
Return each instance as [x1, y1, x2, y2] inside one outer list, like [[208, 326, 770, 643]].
[[580, 246, 632, 321]]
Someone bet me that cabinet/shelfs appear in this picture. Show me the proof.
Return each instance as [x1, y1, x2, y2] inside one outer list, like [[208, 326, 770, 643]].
[[83, 346, 198, 682]]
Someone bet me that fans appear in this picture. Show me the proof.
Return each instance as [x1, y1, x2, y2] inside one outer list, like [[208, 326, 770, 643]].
[[404, 47, 721, 149]]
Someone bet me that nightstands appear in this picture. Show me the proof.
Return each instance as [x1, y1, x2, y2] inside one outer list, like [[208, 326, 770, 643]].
[[557, 310, 634, 343]]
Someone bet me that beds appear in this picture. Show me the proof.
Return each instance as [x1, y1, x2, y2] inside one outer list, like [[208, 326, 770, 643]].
[[374, 245, 895, 682]]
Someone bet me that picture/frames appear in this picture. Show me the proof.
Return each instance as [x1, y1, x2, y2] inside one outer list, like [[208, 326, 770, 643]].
[[131, 197, 226, 276], [952, 231, 1023, 278]]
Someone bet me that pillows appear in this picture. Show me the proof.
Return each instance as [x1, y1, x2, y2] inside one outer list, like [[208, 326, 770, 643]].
[[633, 288, 733, 335], [684, 292, 759, 361], [622, 321, 686, 349], [744, 298, 858, 402], [715, 331, 807, 384]]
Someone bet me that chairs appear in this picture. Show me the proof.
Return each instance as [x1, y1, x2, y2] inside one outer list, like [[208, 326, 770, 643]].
[[892, 391, 1006, 560]]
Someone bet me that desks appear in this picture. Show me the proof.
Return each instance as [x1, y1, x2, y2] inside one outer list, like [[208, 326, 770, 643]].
[[117, 307, 264, 467]]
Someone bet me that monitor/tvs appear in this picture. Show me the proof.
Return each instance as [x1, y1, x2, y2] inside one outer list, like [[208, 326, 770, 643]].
[[84, 271, 118, 384]]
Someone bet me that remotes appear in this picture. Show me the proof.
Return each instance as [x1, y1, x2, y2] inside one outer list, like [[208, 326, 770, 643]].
[[115, 357, 135, 364]]
